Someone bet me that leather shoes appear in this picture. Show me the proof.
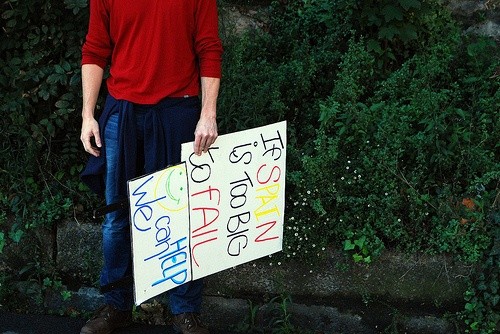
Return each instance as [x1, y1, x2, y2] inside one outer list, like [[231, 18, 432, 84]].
[[78, 303, 133, 334], [173, 312, 210, 334]]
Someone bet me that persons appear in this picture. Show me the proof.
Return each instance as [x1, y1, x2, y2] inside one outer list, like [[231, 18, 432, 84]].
[[80, 0, 222, 334]]
